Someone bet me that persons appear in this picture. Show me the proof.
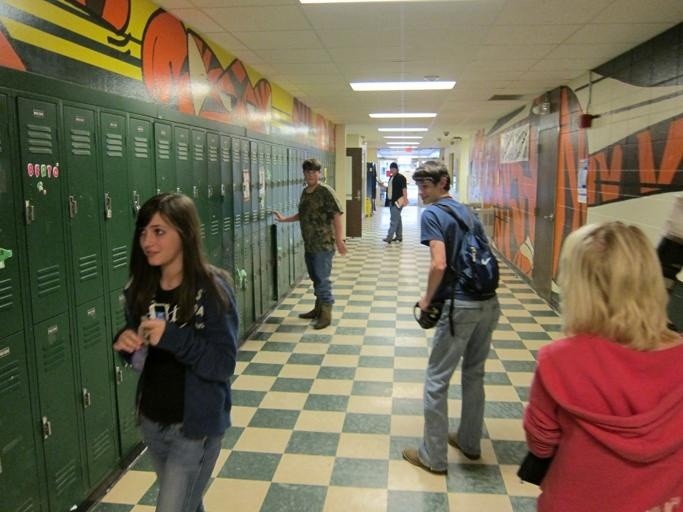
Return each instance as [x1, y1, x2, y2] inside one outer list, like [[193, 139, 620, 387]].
[[270, 157, 348, 331], [654, 232, 681, 332], [111, 191, 241, 512], [376, 161, 408, 245], [400, 159, 502, 476], [520, 218, 682, 512]]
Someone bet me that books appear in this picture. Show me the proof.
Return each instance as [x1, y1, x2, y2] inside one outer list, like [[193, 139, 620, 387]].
[[395, 196, 409, 208]]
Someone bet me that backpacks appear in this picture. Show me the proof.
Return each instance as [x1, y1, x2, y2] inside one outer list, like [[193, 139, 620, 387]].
[[456, 226, 500, 299]]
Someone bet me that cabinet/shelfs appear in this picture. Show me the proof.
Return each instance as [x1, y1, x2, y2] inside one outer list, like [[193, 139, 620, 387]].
[[0, 67, 335, 512]]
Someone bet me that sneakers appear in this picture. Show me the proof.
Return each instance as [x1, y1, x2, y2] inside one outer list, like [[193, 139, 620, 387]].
[[448, 432, 480, 459], [403, 448, 447, 475]]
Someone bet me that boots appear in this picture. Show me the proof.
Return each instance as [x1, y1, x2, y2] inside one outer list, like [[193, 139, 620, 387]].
[[300, 302, 332, 329]]
